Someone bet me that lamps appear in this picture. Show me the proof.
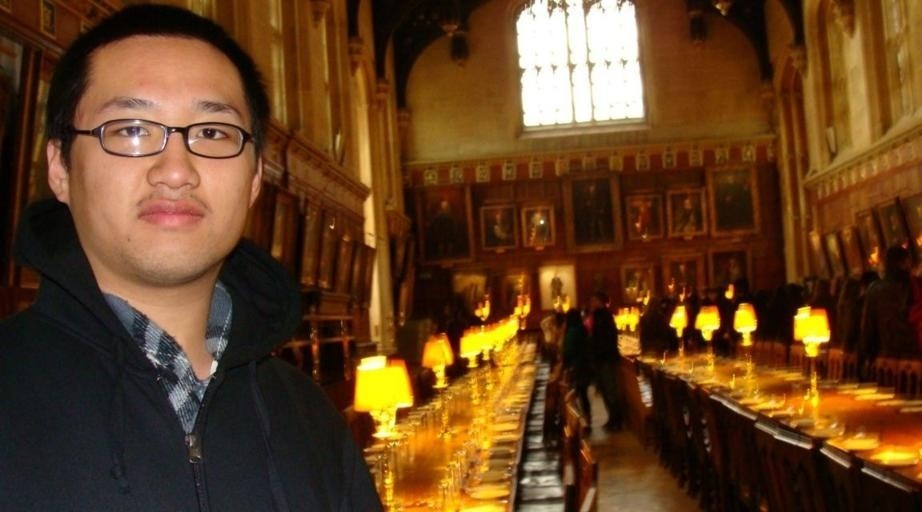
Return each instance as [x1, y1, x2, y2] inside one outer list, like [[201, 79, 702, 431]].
[[421, 317, 519, 438], [354, 313, 524, 439], [616, 305, 831, 399]]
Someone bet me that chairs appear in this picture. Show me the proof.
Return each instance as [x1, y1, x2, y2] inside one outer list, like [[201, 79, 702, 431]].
[[617, 338, 916, 512], [514, 355, 599, 510]]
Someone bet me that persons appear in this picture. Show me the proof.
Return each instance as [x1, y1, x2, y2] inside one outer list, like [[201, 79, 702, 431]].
[[637, 283, 803, 355], [559, 306, 596, 431], [0, 0, 387, 512], [804, 243, 921, 398], [587, 291, 627, 432], [580, 306, 595, 334]]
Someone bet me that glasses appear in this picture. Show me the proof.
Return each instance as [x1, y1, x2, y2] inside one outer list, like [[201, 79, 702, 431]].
[[70, 118, 256, 160]]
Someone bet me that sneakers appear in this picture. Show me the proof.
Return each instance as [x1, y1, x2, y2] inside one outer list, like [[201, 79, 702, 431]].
[[603, 420, 623, 430], [583, 426, 592, 433]]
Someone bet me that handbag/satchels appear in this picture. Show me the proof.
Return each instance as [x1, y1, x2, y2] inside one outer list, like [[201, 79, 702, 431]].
[[907, 304, 922, 352]]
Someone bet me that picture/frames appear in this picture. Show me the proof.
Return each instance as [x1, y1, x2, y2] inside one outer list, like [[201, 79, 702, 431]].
[[706, 166, 763, 237], [499, 271, 534, 312], [665, 187, 707, 237], [626, 188, 667, 240], [538, 263, 580, 313], [454, 268, 493, 310], [563, 170, 622, 256], [480, 204, 518, 253], [0, 0, 60, 289], [660, 252, 705, 294], [707, 244, 754, 287], [620, 260, 653, 299], [415, 184, 477, 267], [242, 181, 377, 387], [807, 190, 922, 285], [521, 205, 556, 249]]
